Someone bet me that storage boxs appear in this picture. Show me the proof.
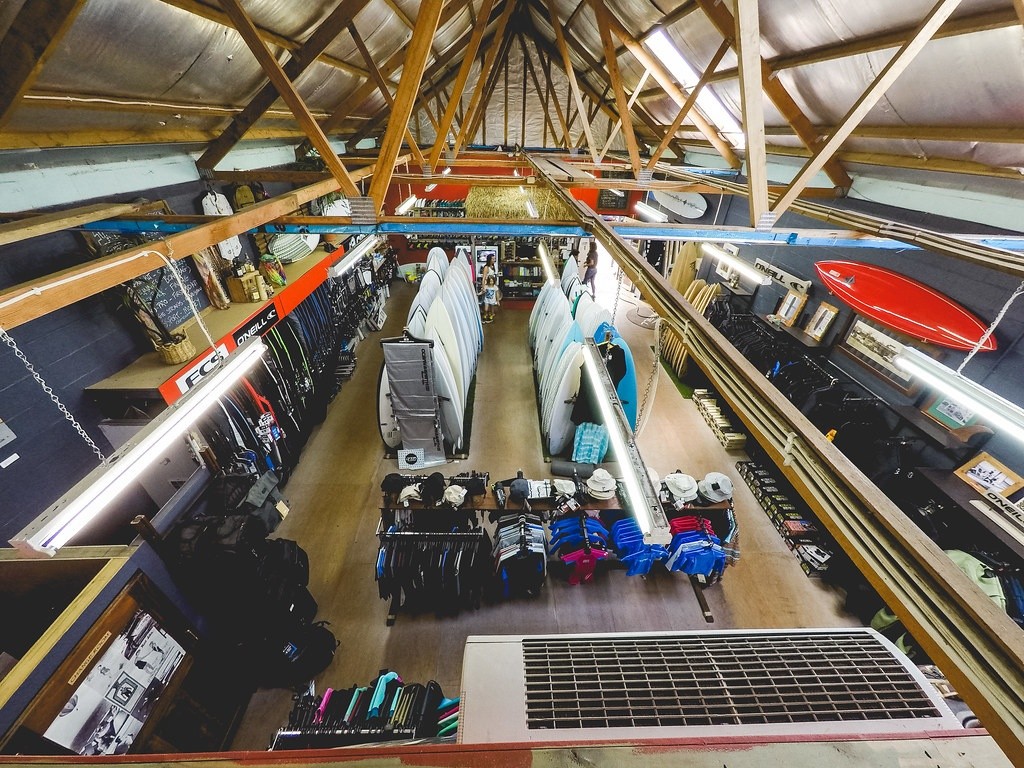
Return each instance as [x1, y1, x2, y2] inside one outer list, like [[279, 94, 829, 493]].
[[736, 460, 834, 579]]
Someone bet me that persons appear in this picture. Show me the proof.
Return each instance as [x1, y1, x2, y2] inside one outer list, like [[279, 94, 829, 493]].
[[481, 253, 498, 306], [582, 242, 598, 302], [563, 249, 580, 269], [476, 275, 500, 320]]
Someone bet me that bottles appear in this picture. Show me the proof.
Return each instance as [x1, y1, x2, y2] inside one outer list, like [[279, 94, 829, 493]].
[[247, 275, 275, 303], [231, 253, 255, 278]]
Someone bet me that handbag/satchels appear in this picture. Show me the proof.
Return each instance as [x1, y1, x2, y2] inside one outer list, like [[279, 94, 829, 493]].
[[238, 470, 294, 532], [214, 514, 263, 544], [175, 512, 217, 559]]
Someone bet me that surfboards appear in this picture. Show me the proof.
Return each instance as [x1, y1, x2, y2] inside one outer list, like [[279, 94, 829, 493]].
[[662, 241, 723, 381], [528, 255, 639, 456], [813, 258, 998, 353], [652, 186, 710, 221], [375, 246, 485, 452]]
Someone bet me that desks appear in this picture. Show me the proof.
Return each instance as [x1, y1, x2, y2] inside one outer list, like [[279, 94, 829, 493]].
[[377, 480, 731, 529]]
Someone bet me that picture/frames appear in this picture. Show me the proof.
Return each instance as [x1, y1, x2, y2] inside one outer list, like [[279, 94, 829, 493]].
[[921, 390, 987, 430], [954, 452, 1024, 500]]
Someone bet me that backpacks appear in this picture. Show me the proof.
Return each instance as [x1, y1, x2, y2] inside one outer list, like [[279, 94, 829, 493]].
[[251, 182, 266, 202], [232, 184, 255, 208]]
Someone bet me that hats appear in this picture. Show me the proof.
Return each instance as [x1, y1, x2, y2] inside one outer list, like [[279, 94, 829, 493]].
[[509, 479, 529, 504], [647, 467, 661, 497], [665, 468, 734, 504], [587, 469, 617, 500], [380, 471, 486, 507]]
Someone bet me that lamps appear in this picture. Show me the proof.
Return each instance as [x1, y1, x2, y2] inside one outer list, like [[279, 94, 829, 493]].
[[0, 327, 268, 559], [699, 208, 793, 285], [890, 284, 1024, 443], [324, 234, 379, 280], [606, 159, 625, 198], [526, 187, 540, 218], [538, 236, 562, 288], [425, 183, 437, 192], [442, 166, 452, 176], [394, 165, 417, 214], [635, 171, 669, 222], [582, 271, 674, 544]]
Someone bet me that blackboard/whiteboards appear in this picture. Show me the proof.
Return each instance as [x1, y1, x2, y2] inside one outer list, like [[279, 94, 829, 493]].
[[73, 200, 217, 341]]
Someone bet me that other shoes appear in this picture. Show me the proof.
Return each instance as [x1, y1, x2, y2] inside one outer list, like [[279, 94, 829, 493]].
[[483, 317, 493, 324]]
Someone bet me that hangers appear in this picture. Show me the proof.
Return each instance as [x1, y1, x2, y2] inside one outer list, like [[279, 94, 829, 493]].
[[750, 323, 776, 344], [895, 436, 917, 447], [730, 312, 750, 324], [799, 355, 839, 386], [513, 510, 533, 554], [714, 293, 730, 305], [696, 512, 715, 550], [206, 184, 215, 195], [842, 394, 876, 410], [278, 710, 417, 742], [579, 519, 592, 553], [382, 531, 479, 552]]
